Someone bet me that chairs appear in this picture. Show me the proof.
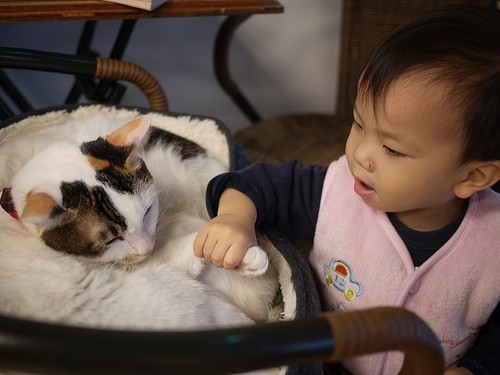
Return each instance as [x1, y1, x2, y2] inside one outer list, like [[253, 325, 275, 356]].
[[0, 48, 444, 375]]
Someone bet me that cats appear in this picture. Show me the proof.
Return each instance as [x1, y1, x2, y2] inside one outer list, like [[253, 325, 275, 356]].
[[0, 113, 279, 331]]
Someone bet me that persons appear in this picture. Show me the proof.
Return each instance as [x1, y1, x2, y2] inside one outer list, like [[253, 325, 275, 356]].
[[194, 5, 500, 375]]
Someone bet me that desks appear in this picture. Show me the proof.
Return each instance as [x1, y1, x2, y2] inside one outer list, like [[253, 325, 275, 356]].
[[0, 0, 284, 123]]
